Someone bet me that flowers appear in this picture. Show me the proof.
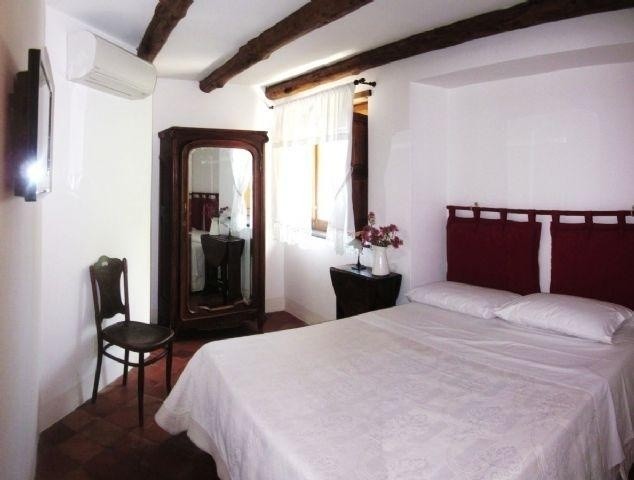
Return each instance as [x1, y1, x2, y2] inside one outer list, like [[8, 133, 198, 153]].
[[354, 211, 406, 250]]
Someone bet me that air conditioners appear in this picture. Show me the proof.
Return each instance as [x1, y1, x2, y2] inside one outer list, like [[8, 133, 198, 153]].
[[68, 28, 157, 101]]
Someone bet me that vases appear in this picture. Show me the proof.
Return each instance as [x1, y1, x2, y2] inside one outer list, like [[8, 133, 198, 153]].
[[371, 245, 390, 277]]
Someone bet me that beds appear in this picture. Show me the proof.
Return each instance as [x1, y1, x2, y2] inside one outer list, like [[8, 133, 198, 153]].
[[189, 192, 219, 292], [188, 206, 634, 479]]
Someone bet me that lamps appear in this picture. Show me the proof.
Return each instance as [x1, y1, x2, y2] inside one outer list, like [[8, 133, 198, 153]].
[[347, 233, 366, 271]]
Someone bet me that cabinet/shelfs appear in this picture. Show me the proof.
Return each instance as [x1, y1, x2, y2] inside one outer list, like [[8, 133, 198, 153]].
[[159, 128, 265, 341]]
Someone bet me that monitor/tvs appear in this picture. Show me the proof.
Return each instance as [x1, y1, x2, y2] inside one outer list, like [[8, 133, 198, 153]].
[[6, 48, 55, 201]]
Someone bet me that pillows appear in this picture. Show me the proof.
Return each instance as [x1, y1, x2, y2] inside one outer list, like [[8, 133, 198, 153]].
[[402, 280, 632, 343]]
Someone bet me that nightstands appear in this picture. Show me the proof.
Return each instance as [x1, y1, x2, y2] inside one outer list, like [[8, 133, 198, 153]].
[[330, 264, 402, 320], [200, 234, 245, 311]]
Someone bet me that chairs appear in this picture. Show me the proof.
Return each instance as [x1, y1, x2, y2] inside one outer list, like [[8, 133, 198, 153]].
[[90, 256, 175, 427]]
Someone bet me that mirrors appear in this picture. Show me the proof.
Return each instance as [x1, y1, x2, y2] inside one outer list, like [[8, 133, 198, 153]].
[[180, 138, 260, 321]]
